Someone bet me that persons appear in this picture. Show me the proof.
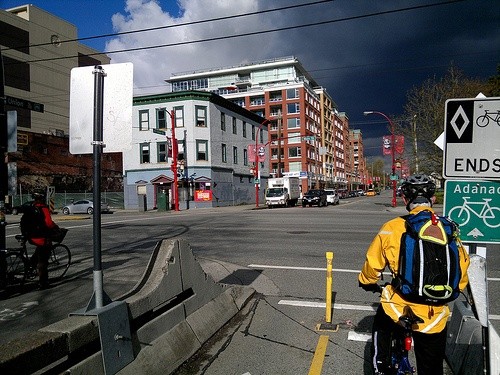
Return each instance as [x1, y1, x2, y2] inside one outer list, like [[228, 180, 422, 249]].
[[26, 189, 59, 289], [358, 173, 469, 375]]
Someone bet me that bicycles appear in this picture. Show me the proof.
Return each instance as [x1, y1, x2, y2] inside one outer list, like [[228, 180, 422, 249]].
[[0, 229, 72, 291], [365, 284, 417, 375]]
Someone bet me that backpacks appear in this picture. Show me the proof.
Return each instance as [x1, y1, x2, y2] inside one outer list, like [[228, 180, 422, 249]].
[[24, 202, 49, 231], [391, 209, 462, 305]]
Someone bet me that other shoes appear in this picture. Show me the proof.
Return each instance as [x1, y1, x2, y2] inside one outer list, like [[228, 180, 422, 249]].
[[40, 284, 50, 289]]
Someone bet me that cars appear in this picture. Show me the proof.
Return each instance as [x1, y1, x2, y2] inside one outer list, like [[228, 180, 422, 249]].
[[12, 201, 49, 215], [367, 189, 380, 196], [340, 189, 364, 199], [62, 199, 109, 215]]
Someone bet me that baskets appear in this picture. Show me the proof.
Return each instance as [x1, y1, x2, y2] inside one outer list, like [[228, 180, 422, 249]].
[[53, 228, 68, 242]]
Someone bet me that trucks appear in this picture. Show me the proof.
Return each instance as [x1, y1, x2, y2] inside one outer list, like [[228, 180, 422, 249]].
[[264, 177, 299, 208]]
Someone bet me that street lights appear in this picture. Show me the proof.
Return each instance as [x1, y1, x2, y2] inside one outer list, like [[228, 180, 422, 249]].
[[364, 111, 396, 207], [255, 115, 274, 207], [155, 107, 179, 211]]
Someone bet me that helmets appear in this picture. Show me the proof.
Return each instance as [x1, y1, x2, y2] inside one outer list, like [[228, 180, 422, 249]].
[[402, 174, 436, 197], [32, 188, 46, 199]]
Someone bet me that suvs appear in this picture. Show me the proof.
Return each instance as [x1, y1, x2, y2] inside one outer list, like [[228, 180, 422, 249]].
[[301, 189, 328, 207], [325, 190, 339, 205]]
